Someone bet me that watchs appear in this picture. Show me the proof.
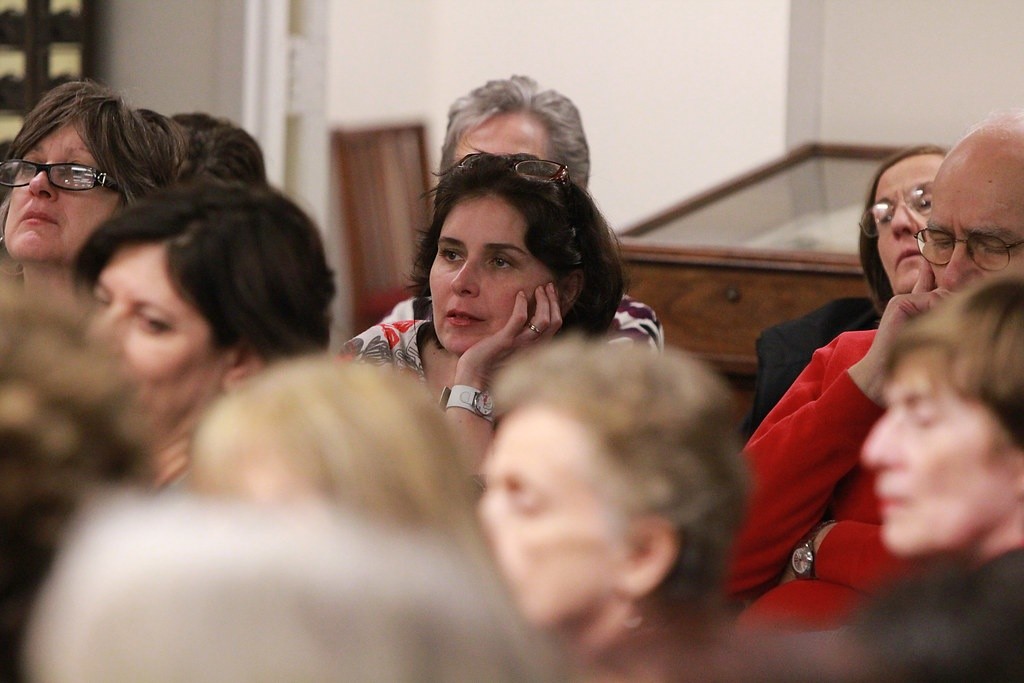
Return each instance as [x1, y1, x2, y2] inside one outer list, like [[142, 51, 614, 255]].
[[792, 519, 839, 582], [438, 385, 495, 421]]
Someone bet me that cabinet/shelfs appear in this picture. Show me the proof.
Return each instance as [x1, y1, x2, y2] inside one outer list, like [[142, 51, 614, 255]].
[[607, 141, 914, 374]]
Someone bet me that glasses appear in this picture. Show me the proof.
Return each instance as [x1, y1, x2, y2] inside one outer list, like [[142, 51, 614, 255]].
[[0, 159, 122, 192], [913, 228, 1024, 271], [858, 186, 932, 237], [456, 154, 570, 185]]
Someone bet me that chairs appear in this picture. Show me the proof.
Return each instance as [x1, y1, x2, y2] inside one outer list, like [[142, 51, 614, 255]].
[[329, 121, 436, 340]]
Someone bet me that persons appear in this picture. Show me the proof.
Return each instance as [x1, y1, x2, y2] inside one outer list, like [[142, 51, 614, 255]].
[[0, 70, 1024, 683]]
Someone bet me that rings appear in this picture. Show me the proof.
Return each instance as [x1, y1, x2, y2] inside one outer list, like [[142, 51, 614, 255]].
[[529, 322, 543, 335]]
